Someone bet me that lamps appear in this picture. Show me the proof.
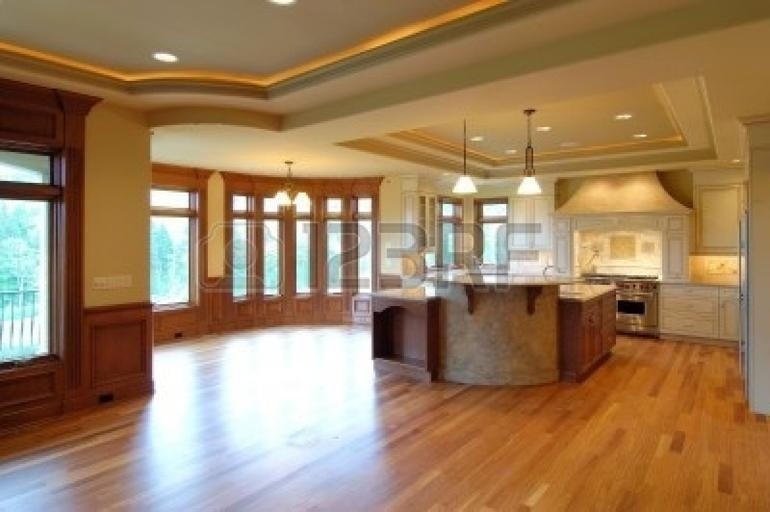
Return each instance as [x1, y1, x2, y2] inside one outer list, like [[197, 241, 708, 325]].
[[516, 109, 542, 195], [451, 121, 477, 194]]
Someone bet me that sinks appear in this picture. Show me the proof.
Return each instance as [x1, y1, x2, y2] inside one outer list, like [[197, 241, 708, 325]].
[[558, 292, 586, 300]]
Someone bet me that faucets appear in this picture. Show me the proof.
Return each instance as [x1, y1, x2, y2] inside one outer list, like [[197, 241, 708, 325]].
[[542, 265, 561, 276], [585, 250, 600, 273]]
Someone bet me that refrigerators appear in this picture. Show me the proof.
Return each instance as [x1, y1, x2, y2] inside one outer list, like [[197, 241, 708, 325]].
[[736, 213, 749, 379]]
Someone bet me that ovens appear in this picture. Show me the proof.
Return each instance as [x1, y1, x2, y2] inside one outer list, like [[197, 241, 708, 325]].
[[613, 279, 657, 327]]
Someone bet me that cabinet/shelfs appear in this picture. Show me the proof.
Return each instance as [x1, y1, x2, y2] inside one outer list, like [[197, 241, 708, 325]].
[[718, 285, 741, 348], [657, 284, 719, 343], [551, 213, 579, 279], [692, 172, 746, 256], [559, 290, 616, 382], [571, 212, 662, 230], [660, 213, 690, 282]]
